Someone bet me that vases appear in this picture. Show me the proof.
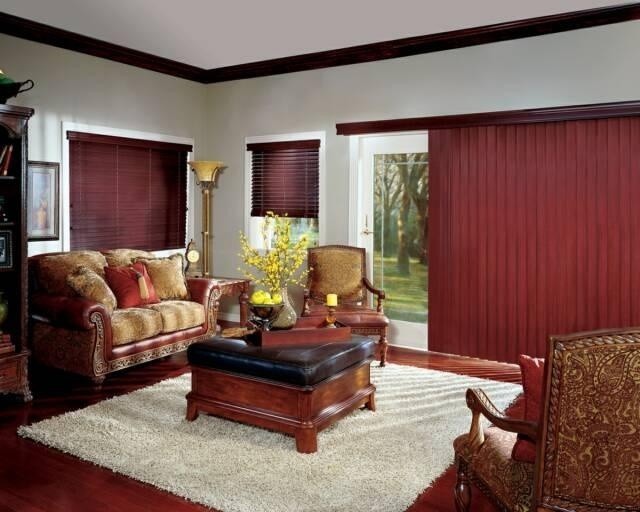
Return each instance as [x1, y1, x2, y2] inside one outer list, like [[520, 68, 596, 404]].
[[269, 288, 297, 330]]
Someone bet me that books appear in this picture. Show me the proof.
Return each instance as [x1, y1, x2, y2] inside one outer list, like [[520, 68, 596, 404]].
[[0, 332, 16, 355], [2, 143, 14, 176], [0, 142, 8, 164]]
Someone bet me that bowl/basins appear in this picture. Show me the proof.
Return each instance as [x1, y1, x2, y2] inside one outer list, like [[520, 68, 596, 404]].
[[250, 304, 285, 318]]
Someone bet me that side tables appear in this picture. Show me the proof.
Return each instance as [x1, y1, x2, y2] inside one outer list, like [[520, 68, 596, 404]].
[[187, 277, 253, 328]]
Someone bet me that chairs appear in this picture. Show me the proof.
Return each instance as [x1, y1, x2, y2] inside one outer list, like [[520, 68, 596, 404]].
[[452, 326, 639, 511], [301, 245, 390, 367]]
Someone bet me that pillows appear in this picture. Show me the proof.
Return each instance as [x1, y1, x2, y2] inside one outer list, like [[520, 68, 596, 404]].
[[65, 265, 118, 310], [28, 250, 109, 296], [106, 248, 155, 268], [510, 353, 548, 465], [133, 252, 192, 301], [103, 261, 161, 309]]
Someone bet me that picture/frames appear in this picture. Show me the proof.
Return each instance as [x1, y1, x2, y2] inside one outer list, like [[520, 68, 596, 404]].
[[27, 160, 59, 241], [0, 231, 13, 269]]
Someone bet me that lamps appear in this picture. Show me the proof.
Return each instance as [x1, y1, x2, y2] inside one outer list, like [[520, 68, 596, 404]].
[[187, 161, 228, 278]]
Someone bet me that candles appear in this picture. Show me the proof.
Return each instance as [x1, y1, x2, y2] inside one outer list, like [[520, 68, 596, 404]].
[[327, 294, 337, 307]]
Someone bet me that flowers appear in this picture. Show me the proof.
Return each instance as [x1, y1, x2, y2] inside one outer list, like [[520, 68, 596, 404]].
[[237, 209, 314, 293]]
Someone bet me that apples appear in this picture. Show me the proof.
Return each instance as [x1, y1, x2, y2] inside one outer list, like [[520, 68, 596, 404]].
[[251, 290, 282, 305]]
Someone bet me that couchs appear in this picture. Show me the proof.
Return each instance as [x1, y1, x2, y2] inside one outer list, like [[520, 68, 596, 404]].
[[27, 248, 222, 393]]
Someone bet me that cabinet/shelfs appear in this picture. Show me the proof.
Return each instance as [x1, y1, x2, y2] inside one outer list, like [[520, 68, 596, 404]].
[[0, 103, 35, 403]]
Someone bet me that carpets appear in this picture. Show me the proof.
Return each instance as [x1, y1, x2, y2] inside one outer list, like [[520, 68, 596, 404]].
[[16, 361, 524, 512]]
